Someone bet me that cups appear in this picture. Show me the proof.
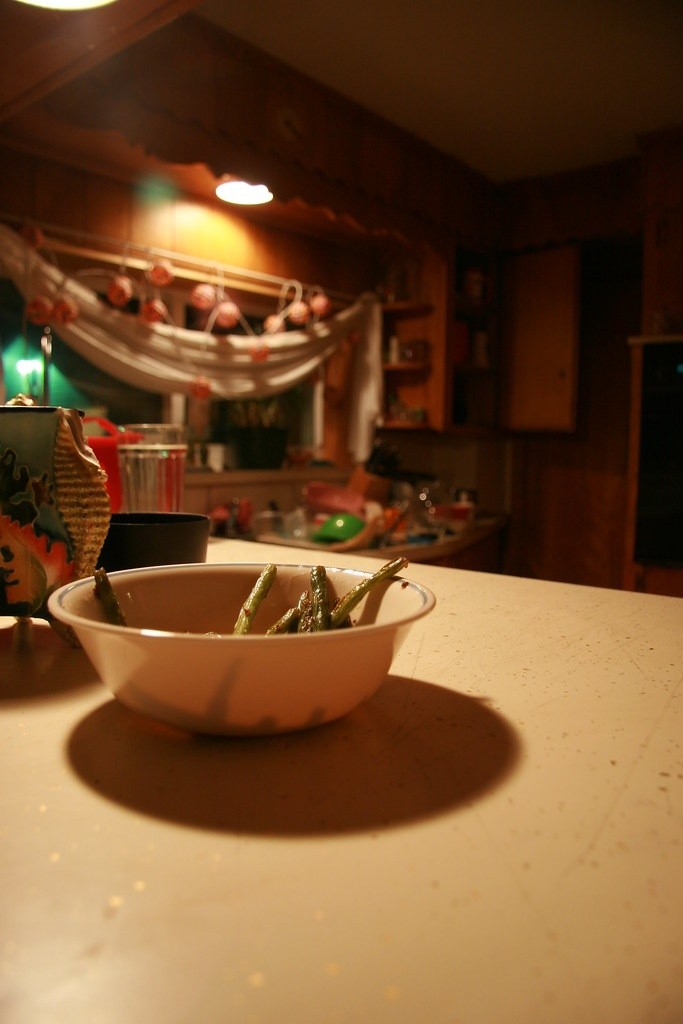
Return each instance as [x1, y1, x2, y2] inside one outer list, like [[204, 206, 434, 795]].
[[114, 423, 187, 513]]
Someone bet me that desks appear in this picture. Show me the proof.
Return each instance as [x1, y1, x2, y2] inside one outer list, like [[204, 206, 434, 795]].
[[0, 540, 683, 1024], [257, 534, 480, 559]]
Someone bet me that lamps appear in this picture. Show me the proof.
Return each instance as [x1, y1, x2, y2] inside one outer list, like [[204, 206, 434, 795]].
[[216, 168, 273, 204]]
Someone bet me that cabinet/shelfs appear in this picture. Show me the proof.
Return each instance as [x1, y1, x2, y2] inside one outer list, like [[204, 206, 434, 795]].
[[384, 239, 579, 433]]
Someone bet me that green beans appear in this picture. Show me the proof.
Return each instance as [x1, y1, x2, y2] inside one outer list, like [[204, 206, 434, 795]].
[[94, 558, 408, 634]]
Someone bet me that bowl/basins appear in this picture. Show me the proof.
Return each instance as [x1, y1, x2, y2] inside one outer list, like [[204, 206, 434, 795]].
[[312, 512, 363, 545], [100, 513, 210, 573], [47, 561, 435, 740]]
